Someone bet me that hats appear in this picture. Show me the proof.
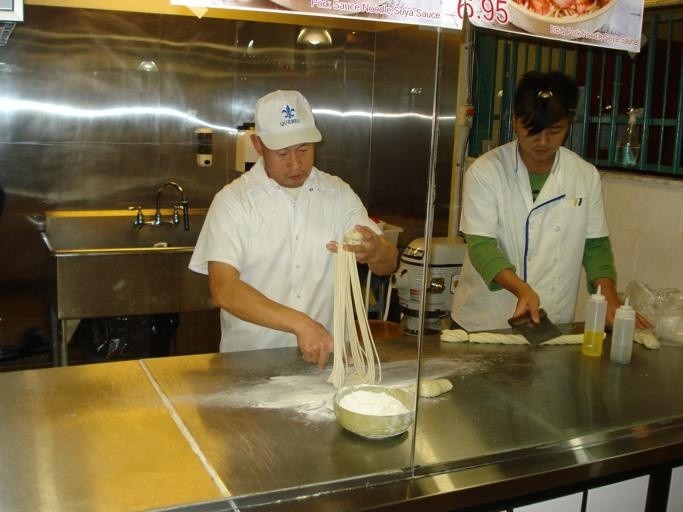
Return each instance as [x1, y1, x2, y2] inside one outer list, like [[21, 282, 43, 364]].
[[253, 88, 322, 150]]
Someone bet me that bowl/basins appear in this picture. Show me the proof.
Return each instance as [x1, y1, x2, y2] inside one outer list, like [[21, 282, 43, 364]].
[[505, 0, 615, 38], [334, 384, 415, 442]]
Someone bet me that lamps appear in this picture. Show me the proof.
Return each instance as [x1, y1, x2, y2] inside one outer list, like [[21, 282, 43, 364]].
[[296, 27, 333, 46]]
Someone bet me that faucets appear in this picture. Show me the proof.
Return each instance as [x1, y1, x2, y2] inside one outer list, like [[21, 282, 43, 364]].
[[153, 181, 190, 231]]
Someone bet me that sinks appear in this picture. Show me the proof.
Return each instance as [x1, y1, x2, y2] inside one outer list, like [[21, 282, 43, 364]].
[[23, 214, 220, 320]]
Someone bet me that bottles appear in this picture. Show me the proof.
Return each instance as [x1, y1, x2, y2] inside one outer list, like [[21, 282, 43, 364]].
[[583, 285, 637, 364]]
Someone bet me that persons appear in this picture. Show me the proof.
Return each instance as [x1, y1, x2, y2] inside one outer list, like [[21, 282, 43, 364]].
[[183, 89, 399, 368], [449, 70, 654, 332]]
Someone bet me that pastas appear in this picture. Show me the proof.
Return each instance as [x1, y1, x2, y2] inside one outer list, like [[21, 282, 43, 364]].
[[326, 241, 382, 390]]
